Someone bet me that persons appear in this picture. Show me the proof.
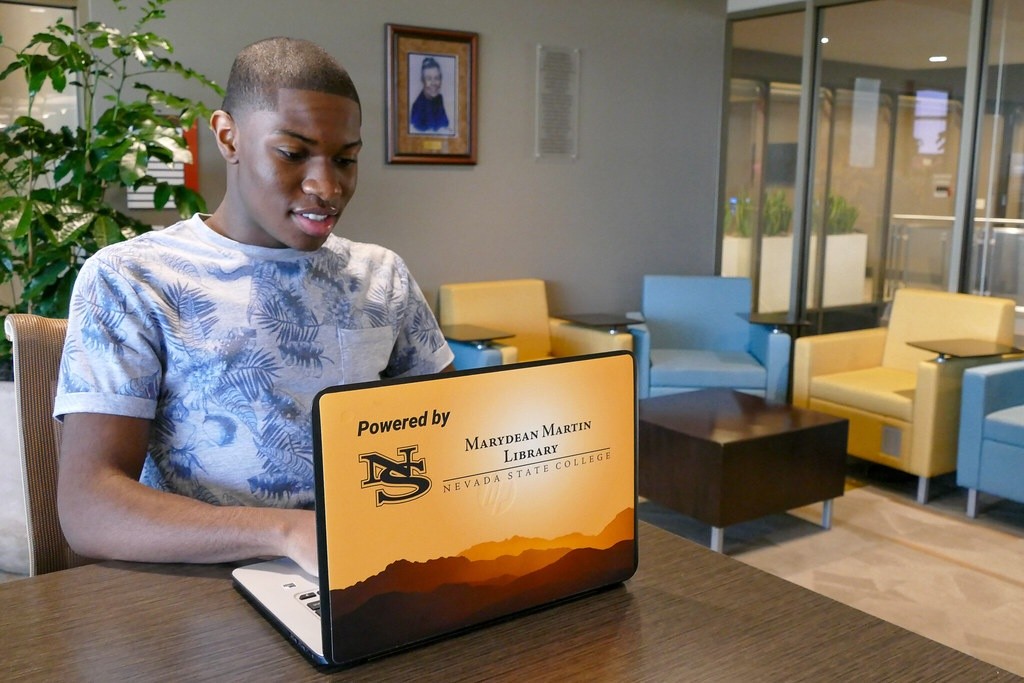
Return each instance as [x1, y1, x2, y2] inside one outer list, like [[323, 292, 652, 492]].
[[52, 36, 455, 577]]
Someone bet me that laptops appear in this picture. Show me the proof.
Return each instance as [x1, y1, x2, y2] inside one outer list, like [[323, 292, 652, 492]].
[[231, 351, 639, 674]]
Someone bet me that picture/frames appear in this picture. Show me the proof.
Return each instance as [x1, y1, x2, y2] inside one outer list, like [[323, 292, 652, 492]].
[[384, 23, 479, 166]]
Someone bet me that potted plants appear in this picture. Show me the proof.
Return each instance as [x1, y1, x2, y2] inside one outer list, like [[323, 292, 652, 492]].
[[805, 188, 868, 307], [0, 0, 227, 572], [720, 186, 795, 314]]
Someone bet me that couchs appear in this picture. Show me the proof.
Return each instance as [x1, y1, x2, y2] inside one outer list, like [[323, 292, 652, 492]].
[[788, 287, 1015, 504], [440, 278, 634, 364], [627, 274, 791, 404]]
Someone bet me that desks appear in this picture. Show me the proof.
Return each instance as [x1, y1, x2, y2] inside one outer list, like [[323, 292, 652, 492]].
[[440, 323, 516, 350], [734, 311, 810, 333], [0, 516, 1024, 683], [906, 339, 1024, 364], [557, 314, 648, 335]]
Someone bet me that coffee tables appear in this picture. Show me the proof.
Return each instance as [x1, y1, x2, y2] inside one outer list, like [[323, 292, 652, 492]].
[[638, 388, 850, 552]]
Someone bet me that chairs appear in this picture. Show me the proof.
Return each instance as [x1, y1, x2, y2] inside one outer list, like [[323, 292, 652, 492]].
[[956, 361, 1023, 518], [4, 314, 91, 577]]
